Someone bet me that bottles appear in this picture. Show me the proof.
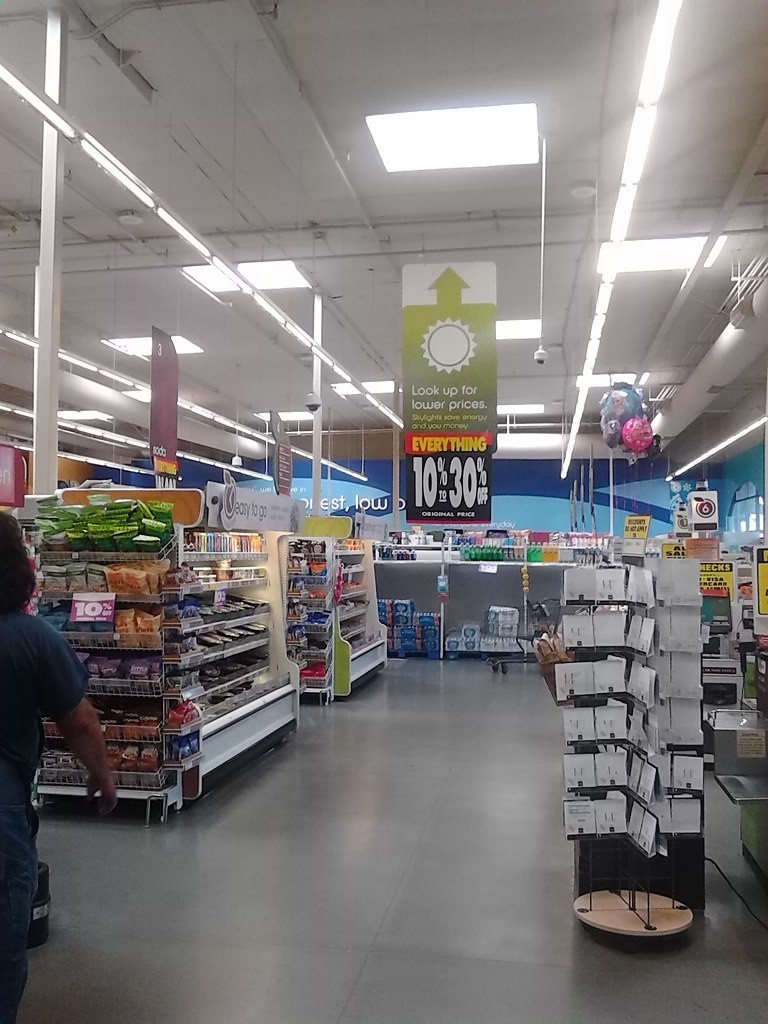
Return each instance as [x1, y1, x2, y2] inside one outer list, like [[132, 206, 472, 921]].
[[372, 530, 663, 651]]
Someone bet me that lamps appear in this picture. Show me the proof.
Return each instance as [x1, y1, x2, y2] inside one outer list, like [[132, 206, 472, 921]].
[[232, 411, 243, 469], [533, 139, 548, 366], [305, 391, 321, 412]]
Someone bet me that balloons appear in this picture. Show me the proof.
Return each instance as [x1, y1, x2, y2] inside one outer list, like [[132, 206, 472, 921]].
[[598, 383, 644, 427], [602, 419, 622, 451], [622, 417, 653, 456]]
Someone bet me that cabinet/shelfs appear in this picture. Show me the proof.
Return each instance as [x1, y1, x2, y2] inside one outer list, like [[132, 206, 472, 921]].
[[331, 539, 388, 696], [32, 523, 182, 823], [288, 537, 335, 705], [179, 526, 300, 805]]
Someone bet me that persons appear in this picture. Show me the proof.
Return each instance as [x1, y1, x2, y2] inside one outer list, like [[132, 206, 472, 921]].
[[0, 511, 116, 1023]]
